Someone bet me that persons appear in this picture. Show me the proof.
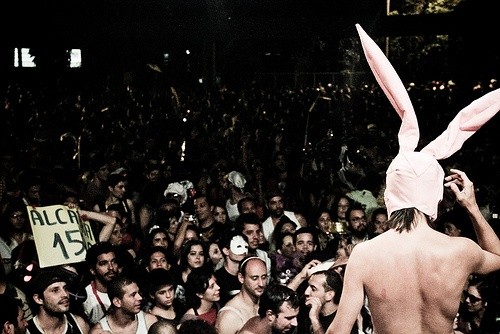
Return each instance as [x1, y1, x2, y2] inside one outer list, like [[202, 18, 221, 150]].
[[214, 255, 268, 334], [176, 266, 221, 331], [286, 259, 374, 334], [23, 270, 90, 334], [0, 253, 34, 321], [84, 240, 120, 325], [0, 295, 29, 334], [143, 268, 189, 326], [236, 283, 300, 334], [178, 319, 216, 334], [407, 79, 500, 334], [0, 63, 402, 307], [148, 320, 178, 334], [88, 275, 157, 334], [323, 151, 500, 334]]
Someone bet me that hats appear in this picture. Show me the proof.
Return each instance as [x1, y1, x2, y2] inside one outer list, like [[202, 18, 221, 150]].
[[164, 182, 187, 206], [356, 23, 500, 221], [225, 171, 247, 193]]
